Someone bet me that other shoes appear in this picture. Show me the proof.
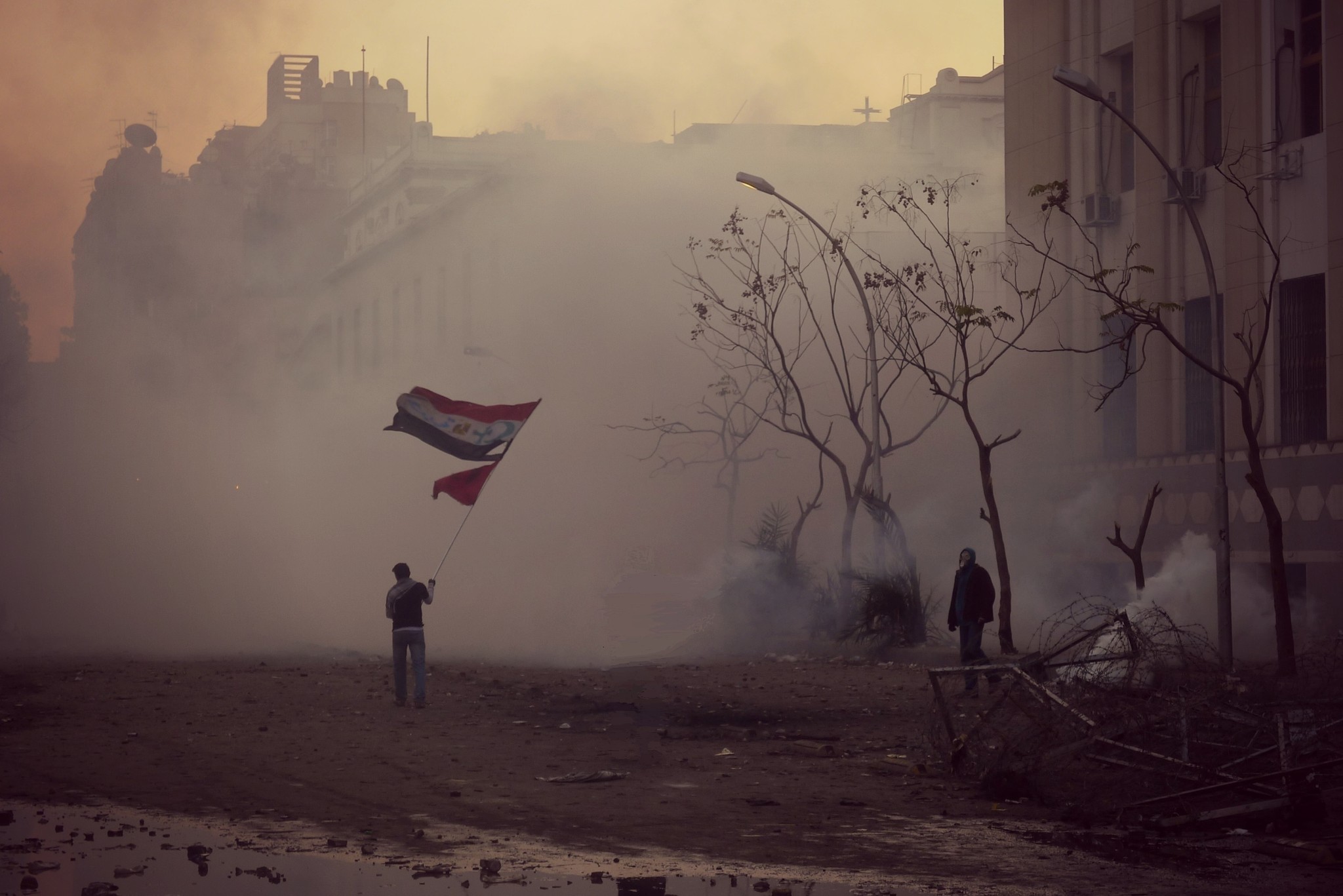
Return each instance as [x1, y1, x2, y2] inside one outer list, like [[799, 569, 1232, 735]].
[[960, 689, 979, 699], [415, 700, 431, 708], [988, 676, 1000, 686], [394, 699, 406, 707]]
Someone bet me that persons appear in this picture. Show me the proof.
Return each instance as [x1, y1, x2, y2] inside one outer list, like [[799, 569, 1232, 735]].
[[947, 548, 999, 698], [385, 562, 435, 709]]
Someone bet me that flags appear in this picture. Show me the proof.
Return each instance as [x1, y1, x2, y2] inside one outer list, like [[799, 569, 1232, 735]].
[[433, 462, 499, 506], [382, 386, 543, 463]]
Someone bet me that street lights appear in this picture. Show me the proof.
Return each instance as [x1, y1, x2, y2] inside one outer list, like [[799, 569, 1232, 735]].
[[1049, 59, 1232, 681], [360, 44, 367, 184], [735, 171, 886, 602]]
